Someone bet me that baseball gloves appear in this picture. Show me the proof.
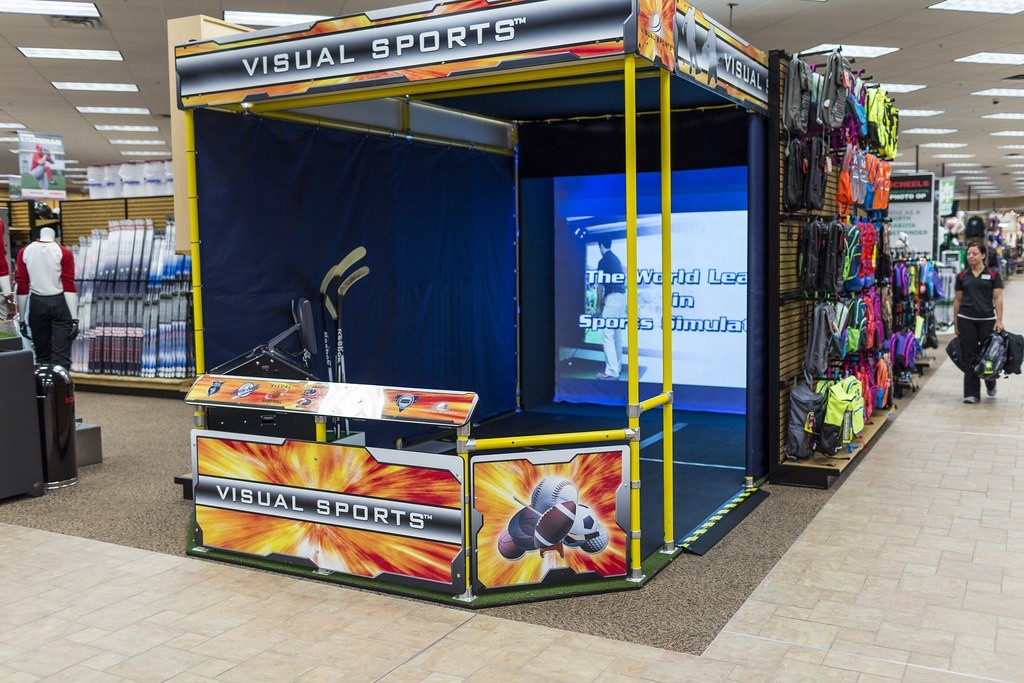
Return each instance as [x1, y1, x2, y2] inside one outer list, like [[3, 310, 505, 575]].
[[44, 149, 51, 156]]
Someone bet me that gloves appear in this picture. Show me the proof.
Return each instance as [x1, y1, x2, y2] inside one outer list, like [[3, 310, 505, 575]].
[[67, 318, 79, 341], [5, 293, 17, 320], [20, 321, 32, 340]]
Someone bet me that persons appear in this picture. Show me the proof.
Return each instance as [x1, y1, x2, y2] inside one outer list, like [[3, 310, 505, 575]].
[[954, 241, 1004, 404], [0, 217, 17, 320], [17, 227, 78, 371], [596, 236, 628, 379], [31, 143, 59, 190]]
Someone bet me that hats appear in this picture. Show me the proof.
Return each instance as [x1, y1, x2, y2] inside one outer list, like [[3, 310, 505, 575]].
[[35, 144, 43, 151]]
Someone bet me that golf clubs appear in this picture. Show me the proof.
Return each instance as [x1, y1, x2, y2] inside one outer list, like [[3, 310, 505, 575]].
[[567, 327, 590, 366]]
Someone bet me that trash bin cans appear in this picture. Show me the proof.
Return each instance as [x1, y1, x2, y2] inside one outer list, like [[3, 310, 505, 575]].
[[35, 364, 82, 491]]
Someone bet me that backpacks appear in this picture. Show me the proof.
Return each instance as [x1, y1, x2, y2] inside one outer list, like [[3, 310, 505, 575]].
[[998, 330, 1024, 375], [782, 45, 947, 463], [973, 335, 1009, 381]]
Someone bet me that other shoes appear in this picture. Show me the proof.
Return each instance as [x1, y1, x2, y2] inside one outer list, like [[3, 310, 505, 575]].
[[596, 369, 619, 382], [987, 384, 997, 396], [49, 179, 57, 185], [963, 396, 977, 403]]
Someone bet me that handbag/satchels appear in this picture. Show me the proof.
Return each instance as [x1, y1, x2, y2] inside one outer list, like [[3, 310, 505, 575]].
[[946, 334, 965, 371]]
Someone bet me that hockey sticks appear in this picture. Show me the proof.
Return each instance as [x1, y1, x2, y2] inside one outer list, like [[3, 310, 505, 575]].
[[318, 246, 370, 438]]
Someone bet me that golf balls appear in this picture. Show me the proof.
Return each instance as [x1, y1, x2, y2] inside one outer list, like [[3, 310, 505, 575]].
[[568, 361, 573, 366]]
